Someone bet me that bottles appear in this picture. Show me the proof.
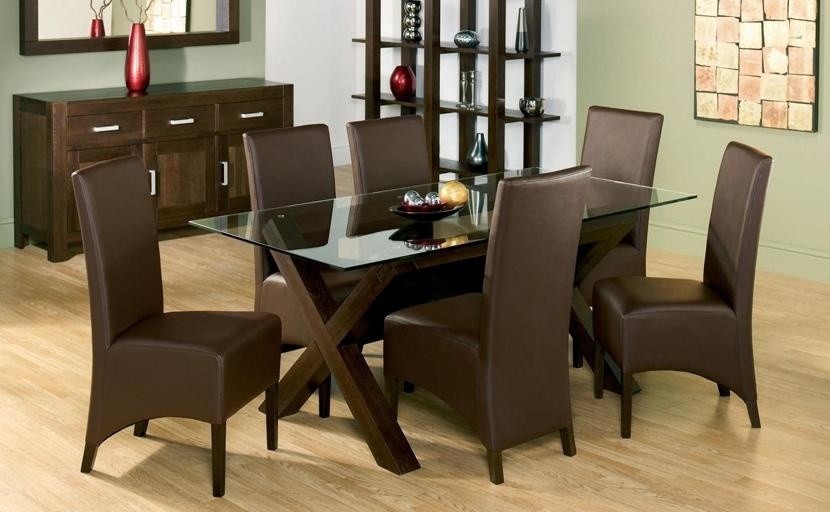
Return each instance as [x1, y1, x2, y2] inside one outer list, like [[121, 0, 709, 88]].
[[390, 65, 417, 101], [460, 71, 475, 109], [466, 188, 484, 226], [466, 133, 488, 171], [514, 8, 530, 54]]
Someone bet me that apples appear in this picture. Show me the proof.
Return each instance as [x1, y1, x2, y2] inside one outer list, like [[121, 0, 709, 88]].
[[439, 181, 468, 209]]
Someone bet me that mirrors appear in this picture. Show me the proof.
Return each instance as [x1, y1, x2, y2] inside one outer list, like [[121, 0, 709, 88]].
[[18, 0, 243, 57]]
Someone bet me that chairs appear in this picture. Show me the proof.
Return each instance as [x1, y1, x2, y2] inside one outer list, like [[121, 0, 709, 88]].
[[370, 161, 598, 487], [559, 101, 668, 379], [343, 117, 434, 199], [584, 138, 777, 443], [240, 122, 417, 420], [71, 148, 287, 497]]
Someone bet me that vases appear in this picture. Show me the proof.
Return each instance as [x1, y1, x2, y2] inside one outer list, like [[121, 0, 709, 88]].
[[89, 18, 105, 39]]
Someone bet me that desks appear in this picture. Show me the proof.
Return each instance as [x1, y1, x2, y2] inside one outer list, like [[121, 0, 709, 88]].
[[181, 163, 703, 476]]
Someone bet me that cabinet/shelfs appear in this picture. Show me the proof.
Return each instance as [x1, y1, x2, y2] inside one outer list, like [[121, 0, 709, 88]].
[[343, 0, 568, 185], [195, 76, 296, 234], [8, 92, 145, 263], [96, 83, 221, 235]]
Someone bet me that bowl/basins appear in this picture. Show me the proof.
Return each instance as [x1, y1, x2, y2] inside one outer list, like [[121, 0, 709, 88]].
[[389, 201, 467, 222], [386, 223, 471, 242], [519, 97, 545, 117]]
